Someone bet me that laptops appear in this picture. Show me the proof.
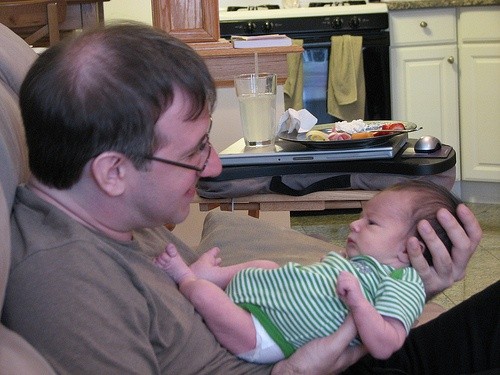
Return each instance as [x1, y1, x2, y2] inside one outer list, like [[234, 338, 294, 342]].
[[218, 133, 409, 166]]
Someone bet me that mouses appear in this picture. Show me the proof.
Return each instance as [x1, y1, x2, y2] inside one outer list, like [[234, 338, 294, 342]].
[[414, 136, 441, 154]]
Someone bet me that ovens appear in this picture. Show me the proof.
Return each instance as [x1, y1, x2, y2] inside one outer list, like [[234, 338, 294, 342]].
[[284, 33, 392, 124]]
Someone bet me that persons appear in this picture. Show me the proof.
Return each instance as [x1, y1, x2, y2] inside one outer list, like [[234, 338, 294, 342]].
[[150, 179, 469, 364], [0, 18, 500, 375]]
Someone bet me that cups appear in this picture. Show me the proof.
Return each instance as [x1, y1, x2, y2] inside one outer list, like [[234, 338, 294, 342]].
[[232, 73, 277, 147]]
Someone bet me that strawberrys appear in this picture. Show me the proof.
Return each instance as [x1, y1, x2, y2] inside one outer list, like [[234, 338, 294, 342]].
[[372, 123, 406, 137]]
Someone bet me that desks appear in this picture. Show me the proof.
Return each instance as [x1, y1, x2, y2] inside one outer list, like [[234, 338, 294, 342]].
[[195, 138, 455, 219]]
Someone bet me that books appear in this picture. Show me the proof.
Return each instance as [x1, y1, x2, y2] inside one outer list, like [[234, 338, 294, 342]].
[[230, 34, 292, 49]]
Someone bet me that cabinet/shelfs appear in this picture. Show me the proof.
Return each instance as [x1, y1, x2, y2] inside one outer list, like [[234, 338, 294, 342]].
[[458, 6, 500, 181], [388, 7, 461, 182]]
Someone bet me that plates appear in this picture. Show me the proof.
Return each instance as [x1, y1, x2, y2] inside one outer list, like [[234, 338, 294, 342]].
[[276, 120, 417, 149]]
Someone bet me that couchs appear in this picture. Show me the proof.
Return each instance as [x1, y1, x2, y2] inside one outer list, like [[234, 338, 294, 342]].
[[1, 25, 343, 375]]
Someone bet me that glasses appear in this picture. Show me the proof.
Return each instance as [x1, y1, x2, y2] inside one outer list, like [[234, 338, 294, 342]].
[[124, 119, 213, 171]]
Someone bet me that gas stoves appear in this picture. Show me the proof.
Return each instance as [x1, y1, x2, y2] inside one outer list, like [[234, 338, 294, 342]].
[[218, 0, 388, 38]]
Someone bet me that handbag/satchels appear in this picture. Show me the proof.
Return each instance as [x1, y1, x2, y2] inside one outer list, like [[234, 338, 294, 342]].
[[195, 167, 456, 199]]
